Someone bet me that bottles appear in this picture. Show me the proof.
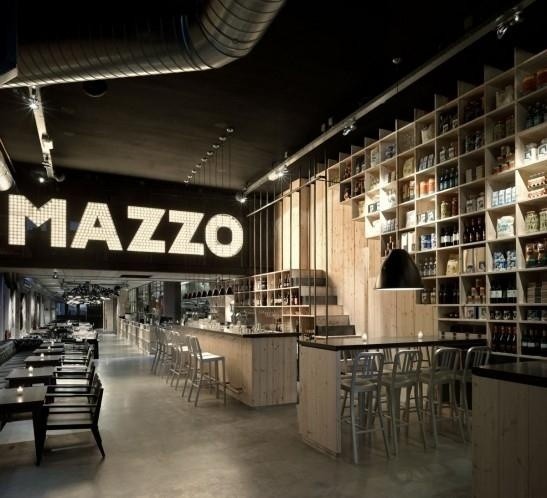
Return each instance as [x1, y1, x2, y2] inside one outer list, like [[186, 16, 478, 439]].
[[440, 215, 485, 246], [439, 279, 458, 304], [279, 274, 290, 288], [271, 291, 299, 305], [492, 324, 547, 357], [440, 166, 458, 191], [526, 101, 546, 128], [424, 256, 437, 276]]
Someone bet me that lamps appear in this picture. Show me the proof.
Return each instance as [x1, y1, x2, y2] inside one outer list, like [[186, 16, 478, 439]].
[[63, 281, 120, 306]]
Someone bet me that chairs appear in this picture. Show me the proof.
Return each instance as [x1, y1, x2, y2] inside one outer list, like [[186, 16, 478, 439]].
[[404, 348, 461, 442], [120, 321, 228, 406], [371, 350, 428, 461], [44, 321, 106, 463], [342, 352, 392, 459]]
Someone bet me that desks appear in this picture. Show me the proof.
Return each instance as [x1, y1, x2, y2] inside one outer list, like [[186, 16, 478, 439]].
[[176, 324, 296, 406], [472, 361, 547, 498], [1, 338, 64, 465], [296, 335, 466, 459]]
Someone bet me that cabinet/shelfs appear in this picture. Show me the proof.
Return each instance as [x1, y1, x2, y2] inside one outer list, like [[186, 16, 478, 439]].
[[271, 3, 547, 371], [181, 268, 356, 334]]
